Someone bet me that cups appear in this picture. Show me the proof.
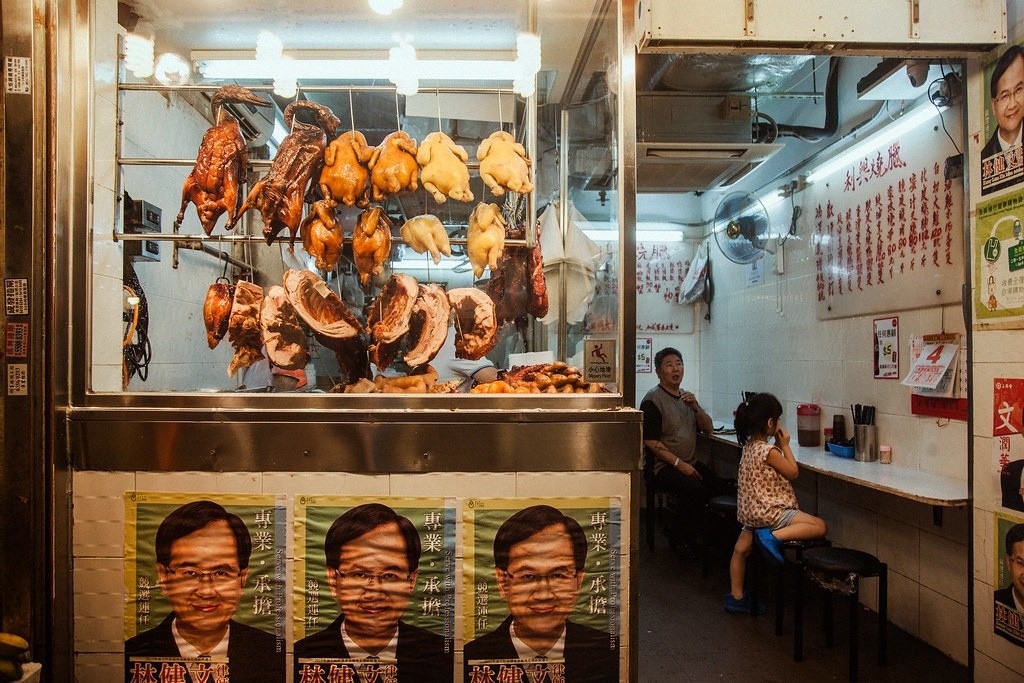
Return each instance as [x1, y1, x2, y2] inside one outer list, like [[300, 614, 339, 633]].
[[854, 424, 876, 462], [833, 415, 846, 444]]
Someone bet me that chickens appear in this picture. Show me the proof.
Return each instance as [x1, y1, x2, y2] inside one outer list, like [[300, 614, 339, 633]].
[[304, 131, 375, 209], [299, 200, 344, 272], [477, 131, 534, 197], [353, 207, 394, 286], [415, 133, 474, 204], [368, 131, 418, 202], [467, 201, 506, 279], [400, 215, 451, 265], [204, 283, 232, 350]]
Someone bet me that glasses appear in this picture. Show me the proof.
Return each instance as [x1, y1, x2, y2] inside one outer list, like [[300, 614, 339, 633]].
[[166, 567, 242, 585], [504, 569, 577, 584], [335, 569, 410, 585]]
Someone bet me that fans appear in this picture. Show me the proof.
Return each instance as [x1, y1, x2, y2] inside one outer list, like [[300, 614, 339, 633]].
[[712, 190, 784, 275]]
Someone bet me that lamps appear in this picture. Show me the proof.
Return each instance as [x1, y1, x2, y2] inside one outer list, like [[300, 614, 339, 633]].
[[582, 230, 685, 242], [191, 49, 519, 81]]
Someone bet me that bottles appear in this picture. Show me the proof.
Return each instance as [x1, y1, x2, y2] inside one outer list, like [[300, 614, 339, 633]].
[[880, 445, 891, 464], [824, 428, 833, 452]]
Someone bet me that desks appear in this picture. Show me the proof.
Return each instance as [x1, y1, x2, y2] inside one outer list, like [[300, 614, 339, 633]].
[[701, 420, 968, 528]]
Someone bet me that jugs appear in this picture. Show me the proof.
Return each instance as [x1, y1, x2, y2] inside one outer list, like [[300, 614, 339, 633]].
[[796, 403, 821, 447]]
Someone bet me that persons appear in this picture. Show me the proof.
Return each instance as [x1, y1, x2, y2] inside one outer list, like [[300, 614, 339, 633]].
[[1000, 407, 1024, 513], [638, 346, 713, 548], [464, 505, 619, 683], [310, 249, 373, 388], [980, 45, 1024, 197], [292, 503, 454, 683], [125, 501, 287, 682], [994, 523, 1024, 650], [721, 392, 826, 613]]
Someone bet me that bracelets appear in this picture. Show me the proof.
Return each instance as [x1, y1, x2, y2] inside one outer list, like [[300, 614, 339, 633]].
[[695, 407, 700, 412], [673, 457, 680, 467]]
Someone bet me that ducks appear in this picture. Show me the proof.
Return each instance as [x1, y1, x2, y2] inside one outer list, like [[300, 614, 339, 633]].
[[177, 85, 273, 236], [232, 100, 341, 254]]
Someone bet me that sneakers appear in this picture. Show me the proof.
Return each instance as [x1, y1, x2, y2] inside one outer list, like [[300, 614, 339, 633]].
[[724, 594, 768, 616], [754, 528, 785, 564]]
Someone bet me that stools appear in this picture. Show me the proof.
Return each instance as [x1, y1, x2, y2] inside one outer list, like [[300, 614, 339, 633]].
[[644, 480, 832, 644], [792, 546, 888, 683]]
